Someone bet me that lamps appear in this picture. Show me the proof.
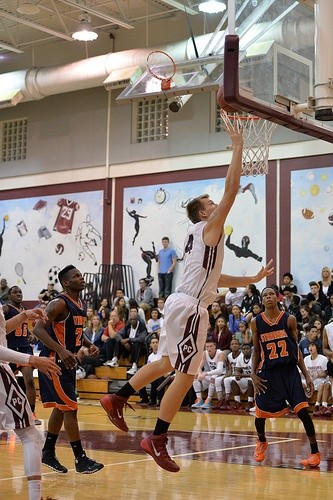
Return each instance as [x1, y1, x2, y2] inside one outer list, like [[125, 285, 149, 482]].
[[72, 0, 98, 41], [199, 0, 227, 14]]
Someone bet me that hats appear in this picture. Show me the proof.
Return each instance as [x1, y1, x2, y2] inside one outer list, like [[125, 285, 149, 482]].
[[48, 281, 55, 284], [309, 281, 320, 288]]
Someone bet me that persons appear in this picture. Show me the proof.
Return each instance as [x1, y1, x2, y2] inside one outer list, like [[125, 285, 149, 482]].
[[99, 120, 245, 473], [157, 235, 177, 301], [248, 285, 321, 467], [182, 267, 333, 416], [31, 264, 104, 474], [0, 300, 63, 500], [36, 278, 174, 408], [1, 285, 44, 425], [0, 278, 11, 303]]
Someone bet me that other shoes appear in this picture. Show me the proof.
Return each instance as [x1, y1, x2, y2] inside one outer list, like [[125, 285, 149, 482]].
[[250, 405, 257, 411], [147, 400, 157, 405], [33, 413, 41, 425], [137, 398, 150, 404]]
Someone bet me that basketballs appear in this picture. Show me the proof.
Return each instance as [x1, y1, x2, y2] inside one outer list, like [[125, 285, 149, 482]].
[[217, 83, 241, 113]]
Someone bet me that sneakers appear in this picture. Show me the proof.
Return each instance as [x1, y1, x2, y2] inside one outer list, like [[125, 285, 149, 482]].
[[140, 433, 180, 472], [313, 405, 328, 417], [75, 453, 104, 474], [100, 393, 135, 433], [325, 407, 333, 417], [229, 401, 242, 410], [126, 368, 137, 375], [41, 449, 68, 473], [191, 398, 203, 407], [106, 361, 118, 368], [211, 399, 231, 410], [244, 402, 254, 412], [201, 399, 214, 408], [301, 452, 321, 467], [254, 439, 269, 462]]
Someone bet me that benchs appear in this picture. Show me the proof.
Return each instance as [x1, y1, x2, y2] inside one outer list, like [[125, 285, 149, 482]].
[[34, 349, 333, 408]]
